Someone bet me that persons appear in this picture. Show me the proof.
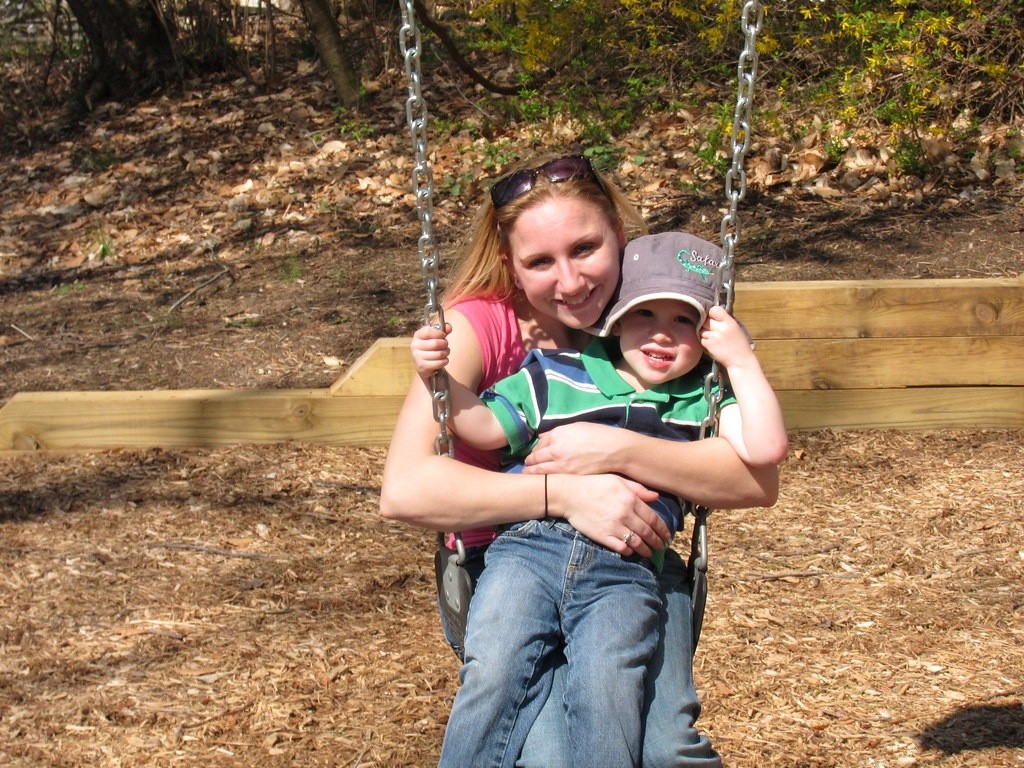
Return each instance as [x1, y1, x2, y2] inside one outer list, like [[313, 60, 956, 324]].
[[380, 152, 788, 767]]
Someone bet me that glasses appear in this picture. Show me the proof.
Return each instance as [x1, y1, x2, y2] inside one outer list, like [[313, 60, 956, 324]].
[[488, 153, 616, 210]]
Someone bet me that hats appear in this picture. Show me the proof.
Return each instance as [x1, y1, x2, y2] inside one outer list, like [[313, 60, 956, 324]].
[[581, 231, 756, 350]]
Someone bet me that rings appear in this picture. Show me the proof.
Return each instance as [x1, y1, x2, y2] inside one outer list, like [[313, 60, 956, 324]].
[[624, 531, 634, 545]]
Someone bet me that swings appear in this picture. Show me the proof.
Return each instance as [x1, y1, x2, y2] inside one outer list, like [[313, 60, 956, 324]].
[[390, 0, 773, 669]]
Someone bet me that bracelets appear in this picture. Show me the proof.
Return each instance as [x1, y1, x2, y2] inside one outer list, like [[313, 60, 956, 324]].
[[545, 474, 548, 521]]
